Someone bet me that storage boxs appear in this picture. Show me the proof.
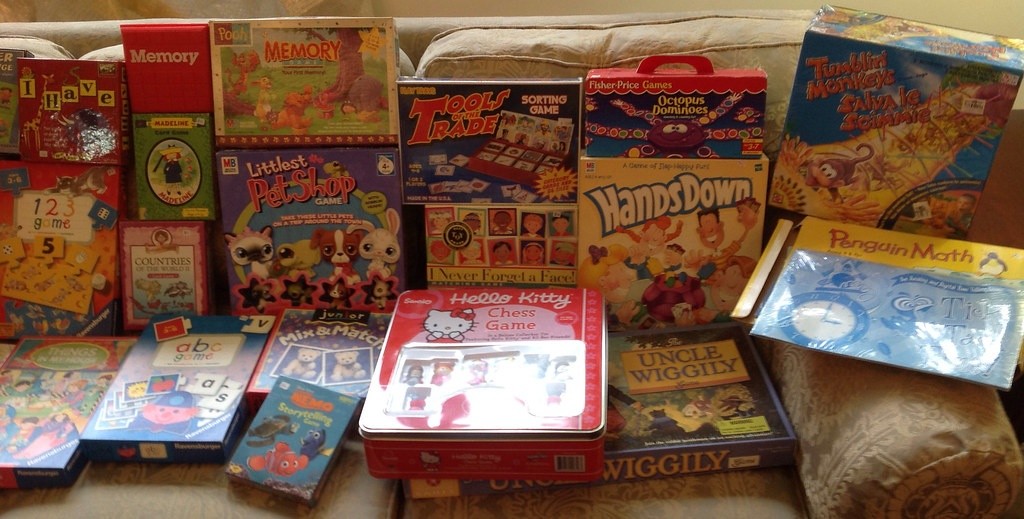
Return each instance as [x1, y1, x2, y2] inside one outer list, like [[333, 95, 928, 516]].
[[0, 6, 1024, 508]]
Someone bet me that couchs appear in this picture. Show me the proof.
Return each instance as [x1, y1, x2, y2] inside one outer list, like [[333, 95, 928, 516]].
[[0, 9, 1024, 519]]
